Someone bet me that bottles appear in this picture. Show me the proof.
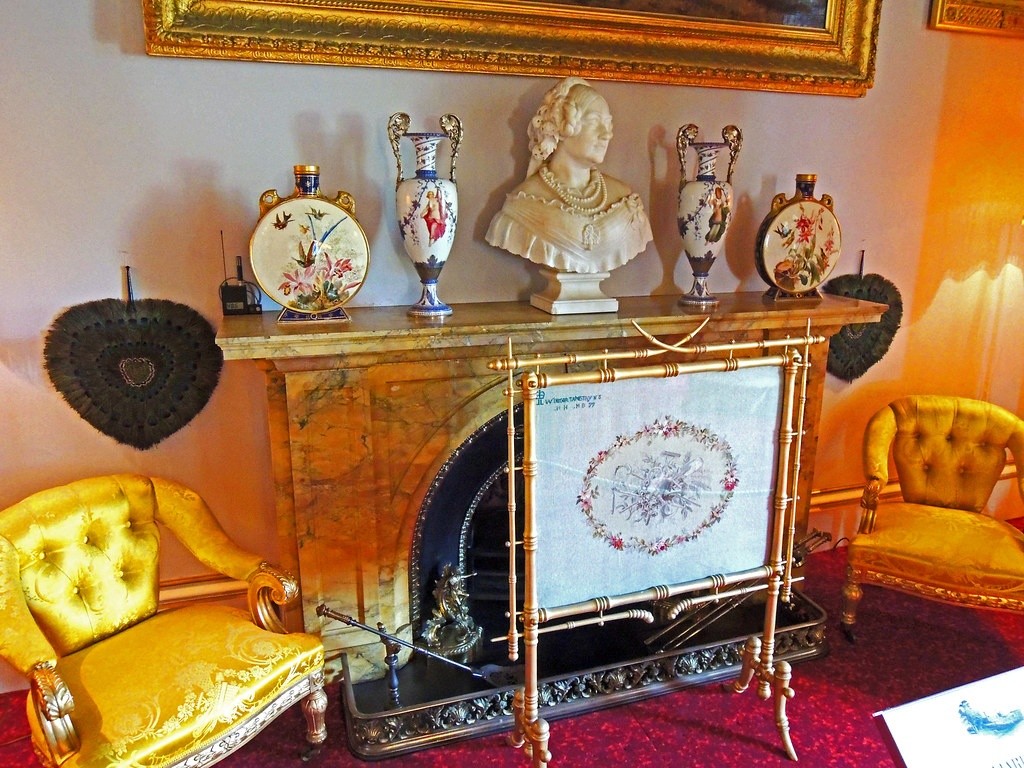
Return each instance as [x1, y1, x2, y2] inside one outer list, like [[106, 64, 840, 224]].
[[677, 122, 743, 305], [250, 164, 370, 323], [754, 173, 843, 302], [386, 112, 463, 316]]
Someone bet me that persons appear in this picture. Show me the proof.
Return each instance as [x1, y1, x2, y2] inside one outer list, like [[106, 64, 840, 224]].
[[485, 74, 654, 274]]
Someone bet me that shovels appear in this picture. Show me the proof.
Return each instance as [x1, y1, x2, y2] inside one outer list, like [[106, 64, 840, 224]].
[[316, 603, 525, 687]]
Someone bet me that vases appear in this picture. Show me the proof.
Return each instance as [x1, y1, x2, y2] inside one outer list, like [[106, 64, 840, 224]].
[[387, 112, 463, 316], [676, 124, 743, 308]]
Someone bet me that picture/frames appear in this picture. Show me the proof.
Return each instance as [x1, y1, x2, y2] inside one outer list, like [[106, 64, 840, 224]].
[[142, 1, 883, 98]]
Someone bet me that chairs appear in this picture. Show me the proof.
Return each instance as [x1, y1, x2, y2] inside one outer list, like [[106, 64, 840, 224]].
[[839, 395, 1024, 637], [0, 474, 329, 768]]
